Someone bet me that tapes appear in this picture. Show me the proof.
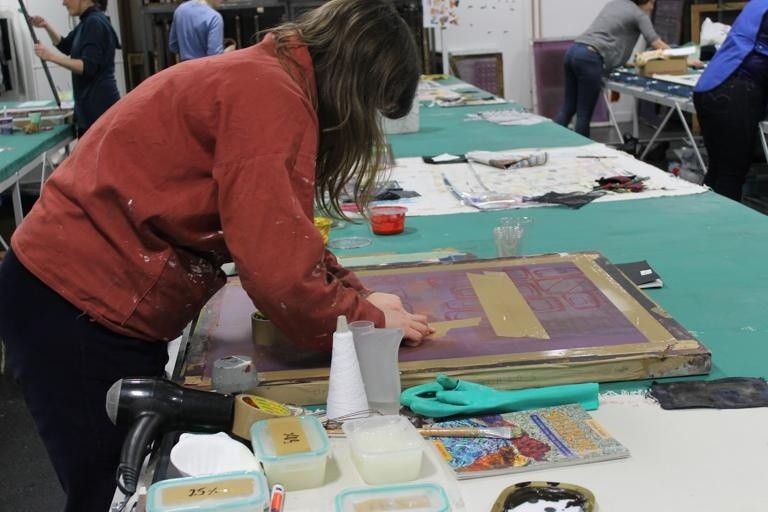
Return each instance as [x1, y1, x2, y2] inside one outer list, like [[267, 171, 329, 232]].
[[251, 310, 291, 347], [231, 393, 293, 443]]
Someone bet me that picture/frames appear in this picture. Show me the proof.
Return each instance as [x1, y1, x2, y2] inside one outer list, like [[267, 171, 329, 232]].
[[448, 52, 504, 98], [529, 37, 614, 127]]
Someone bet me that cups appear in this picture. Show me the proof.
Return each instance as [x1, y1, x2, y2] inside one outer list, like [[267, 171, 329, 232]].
[[494, 216, 532, 257], [0, 117, 14, 133], [28, 112, 41, 133]]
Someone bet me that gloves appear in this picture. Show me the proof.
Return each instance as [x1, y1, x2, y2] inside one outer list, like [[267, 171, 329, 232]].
[[399, 374, 600, 418]]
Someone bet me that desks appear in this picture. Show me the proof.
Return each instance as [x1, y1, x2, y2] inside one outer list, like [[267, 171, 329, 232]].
[[106, 74, 768, 512], [0, 99, 79, 256], [601, 67, 768, 207]]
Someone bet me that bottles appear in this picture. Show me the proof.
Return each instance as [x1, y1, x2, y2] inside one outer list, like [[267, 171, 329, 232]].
[[351, 321, 405, 414]]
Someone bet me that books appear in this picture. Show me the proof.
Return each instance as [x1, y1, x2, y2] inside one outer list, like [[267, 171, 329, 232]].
[[422, 403, 631, 480]]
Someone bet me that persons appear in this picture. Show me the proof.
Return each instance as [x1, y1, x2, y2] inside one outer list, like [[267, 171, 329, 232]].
[[553, 0, 672, 139], [26, 1, 120, 139], [169, 1, 236, 62], [693, 1, 767, 203], [1, 1, 437, 510]]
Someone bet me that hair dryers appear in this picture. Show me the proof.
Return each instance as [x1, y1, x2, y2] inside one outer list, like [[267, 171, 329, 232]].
[[106, 378, 234, 495]]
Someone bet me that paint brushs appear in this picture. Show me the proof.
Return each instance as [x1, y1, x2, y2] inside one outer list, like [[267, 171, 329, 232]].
[[326, 426, 523, 440]]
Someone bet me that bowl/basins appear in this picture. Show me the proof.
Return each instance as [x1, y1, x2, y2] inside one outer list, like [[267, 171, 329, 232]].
[[168, 431, 257, 478], [370, 206, 408, 235]]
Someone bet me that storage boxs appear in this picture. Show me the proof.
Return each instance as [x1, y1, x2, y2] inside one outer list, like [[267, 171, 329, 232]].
[[639, 56, 689, 77]]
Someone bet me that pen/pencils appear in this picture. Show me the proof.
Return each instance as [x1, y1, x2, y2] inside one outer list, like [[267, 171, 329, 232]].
[[270, 484, 285, 512]]
